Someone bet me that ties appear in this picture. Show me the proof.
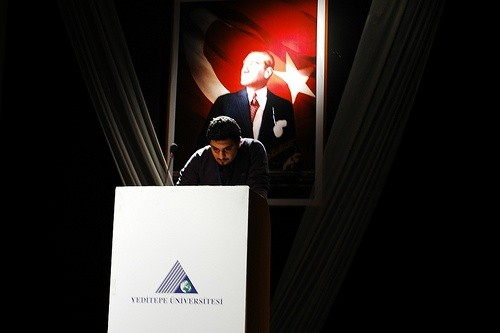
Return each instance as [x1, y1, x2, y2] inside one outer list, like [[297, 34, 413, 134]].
[[250, 96, 260, 124]]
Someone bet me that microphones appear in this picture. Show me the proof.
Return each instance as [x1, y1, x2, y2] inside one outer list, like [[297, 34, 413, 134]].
[[163, 143, 178, 186]]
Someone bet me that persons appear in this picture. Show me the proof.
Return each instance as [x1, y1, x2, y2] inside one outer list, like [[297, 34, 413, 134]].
[[204, 50, 298, 170], [175, 116, 269, 198]]
